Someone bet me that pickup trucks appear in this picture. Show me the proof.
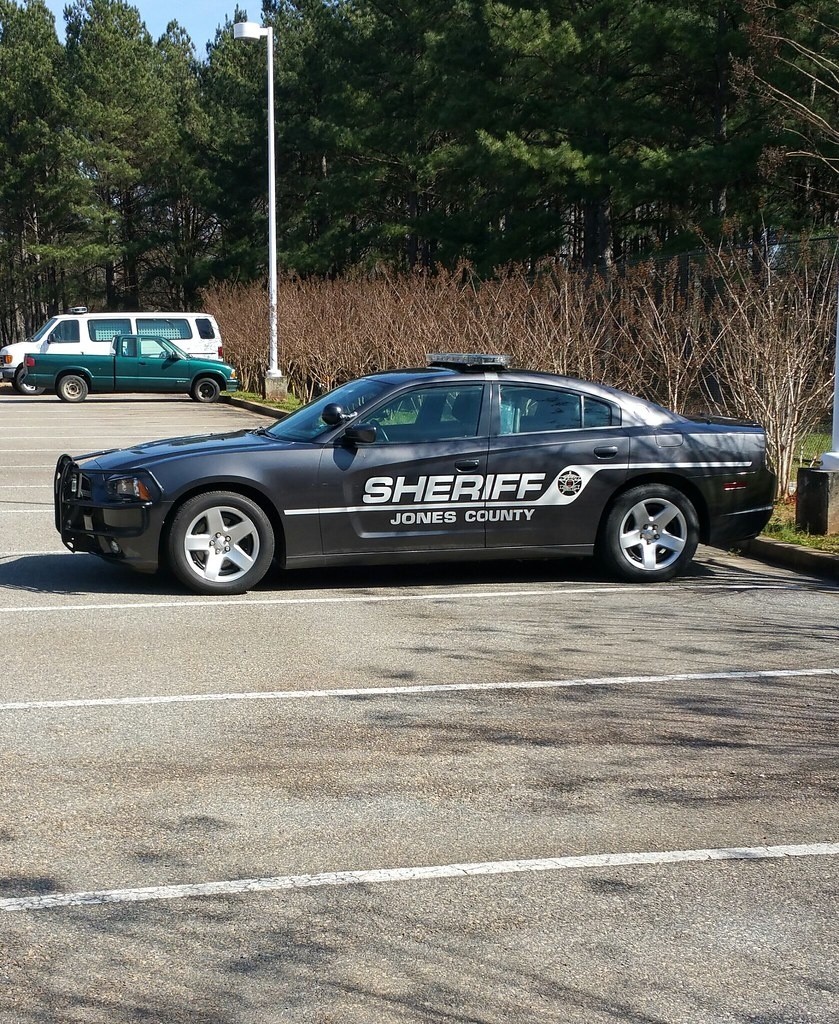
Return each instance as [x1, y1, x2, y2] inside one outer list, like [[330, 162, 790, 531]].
[[23, 335, 240, 403]]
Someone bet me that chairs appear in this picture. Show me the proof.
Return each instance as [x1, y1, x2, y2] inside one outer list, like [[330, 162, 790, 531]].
[[520, 399, 579, 431], [437, 390, 482, 436]]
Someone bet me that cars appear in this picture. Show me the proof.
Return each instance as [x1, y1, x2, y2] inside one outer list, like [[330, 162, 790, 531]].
[[54, 353, 779, 596]]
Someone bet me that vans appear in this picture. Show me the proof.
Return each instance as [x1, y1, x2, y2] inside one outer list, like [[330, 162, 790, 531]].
[[0, 305, 225, 396]]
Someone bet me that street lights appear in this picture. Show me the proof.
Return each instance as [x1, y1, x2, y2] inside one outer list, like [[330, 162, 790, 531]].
[[231, 22, 290, 404]]
